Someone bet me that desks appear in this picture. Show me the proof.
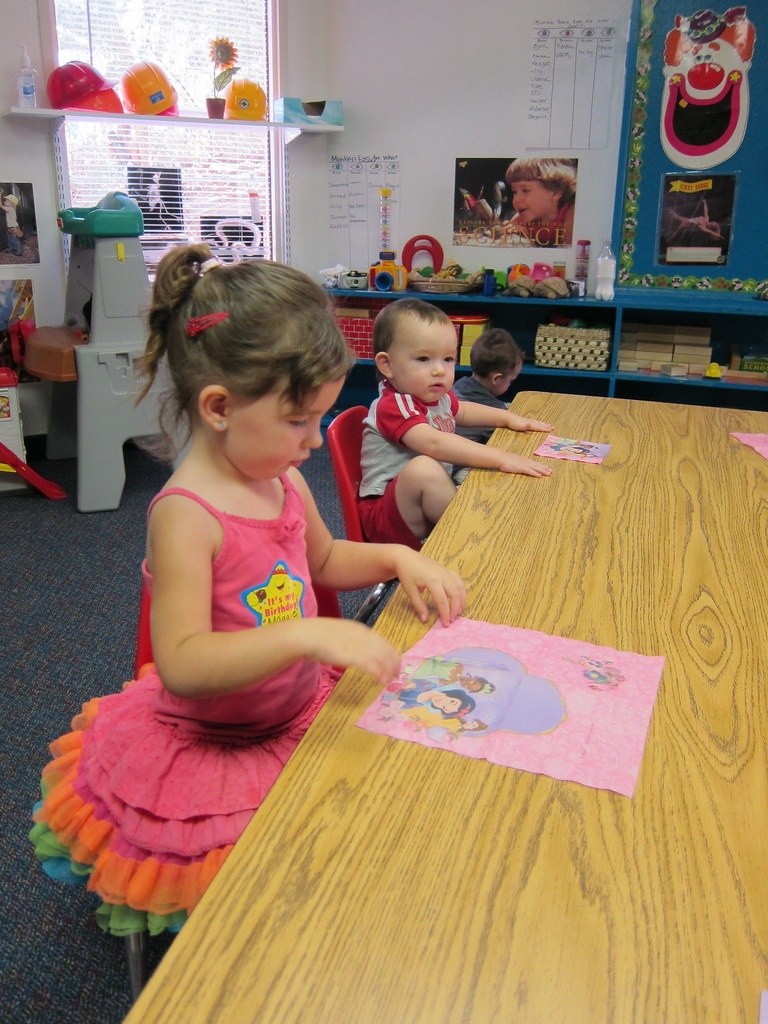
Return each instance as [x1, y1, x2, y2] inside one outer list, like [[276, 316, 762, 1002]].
[[122, 392, 768, 1024]]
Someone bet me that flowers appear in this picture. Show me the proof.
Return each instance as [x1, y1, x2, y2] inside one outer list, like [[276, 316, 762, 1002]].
[[210, 36, 241, 99]]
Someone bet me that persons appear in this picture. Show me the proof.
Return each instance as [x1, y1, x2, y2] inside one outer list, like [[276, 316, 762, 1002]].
[[0, 194, 24, 257], [352, 297, 555, 551], [490, 157, 578, 249], [664, 195, 727, 249], [448, 327, 524, 486], [35, 257, 469, 932]]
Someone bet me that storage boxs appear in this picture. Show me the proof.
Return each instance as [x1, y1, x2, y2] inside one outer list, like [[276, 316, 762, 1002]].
[[273, 97, 345, 125]]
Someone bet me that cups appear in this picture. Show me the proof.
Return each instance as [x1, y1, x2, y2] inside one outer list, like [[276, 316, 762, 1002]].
[[553, 261, 566, 277]]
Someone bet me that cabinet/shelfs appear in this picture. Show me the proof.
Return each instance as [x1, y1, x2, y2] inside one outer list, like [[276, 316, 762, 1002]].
[[330, 287, 768, 398]]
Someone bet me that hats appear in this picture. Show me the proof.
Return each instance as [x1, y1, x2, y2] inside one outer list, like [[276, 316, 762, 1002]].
[[5, 195, 18, 204]]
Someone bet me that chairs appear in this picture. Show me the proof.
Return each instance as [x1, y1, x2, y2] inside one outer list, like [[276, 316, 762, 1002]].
[[328, 406, 396, 626]]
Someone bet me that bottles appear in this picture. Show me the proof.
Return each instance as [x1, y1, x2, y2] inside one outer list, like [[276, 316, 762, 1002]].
[[595, 240, 616, 300], [484, 268, 495, 295]]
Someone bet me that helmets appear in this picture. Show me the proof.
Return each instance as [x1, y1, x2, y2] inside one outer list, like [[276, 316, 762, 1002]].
[[160, 104, 181, 116], [123, 62, 177, 113], [72, 89, 124, 113], [47, 61, 117, 108], [227, 80, 266, 120]]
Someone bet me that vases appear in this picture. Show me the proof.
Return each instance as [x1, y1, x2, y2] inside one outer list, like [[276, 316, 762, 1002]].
[[207, 98, 226, 119]]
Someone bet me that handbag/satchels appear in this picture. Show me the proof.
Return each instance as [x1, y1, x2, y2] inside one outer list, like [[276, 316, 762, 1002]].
[[14, 227, 23, 237]]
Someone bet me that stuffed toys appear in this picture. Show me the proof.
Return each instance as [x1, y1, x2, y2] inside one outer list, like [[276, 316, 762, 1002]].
[[530, 277, 570, 299], [503, 275, 537, 298]]
